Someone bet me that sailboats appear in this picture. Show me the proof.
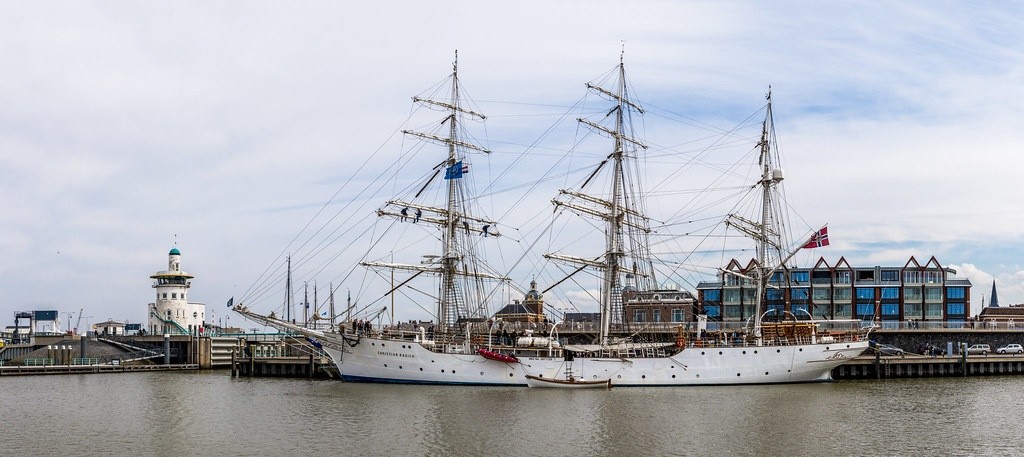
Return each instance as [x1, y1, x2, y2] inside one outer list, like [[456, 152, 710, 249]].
[[225, 47, 870, 391]]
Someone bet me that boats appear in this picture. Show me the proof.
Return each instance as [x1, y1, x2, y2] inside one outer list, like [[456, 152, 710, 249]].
[[525, 374, 613, 389]]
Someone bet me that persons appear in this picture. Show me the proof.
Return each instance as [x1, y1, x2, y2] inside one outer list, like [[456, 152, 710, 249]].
[[543, 317, 552, 330], [496, 329, 525, 347], [499, 321, 504, 332], [352, 319, 373, 337], [908, 317, 918, 329], [700, 328, 707, 347], [463, 222, 470, 235], [924, 343, 933, 356], [400, 208, 408, 222], [413, 209, 421, 223], [970, 317, 975, 330], [479, 225, 490, 237]]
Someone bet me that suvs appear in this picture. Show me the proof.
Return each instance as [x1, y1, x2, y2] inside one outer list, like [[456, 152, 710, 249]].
[[874, 344, 905, 356], [960, 343, 991, 355]]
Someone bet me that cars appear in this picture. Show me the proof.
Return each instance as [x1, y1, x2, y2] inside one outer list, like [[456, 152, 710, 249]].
[[920, 345, 948, 355], [996, 343, 1024, 355]]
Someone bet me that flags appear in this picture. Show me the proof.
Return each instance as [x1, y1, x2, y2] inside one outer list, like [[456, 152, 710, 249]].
[[227, 298, 234, 307], [463, 166, 468, 174], [445, 161, 462, 178], [800, 227, 829, 248]]
